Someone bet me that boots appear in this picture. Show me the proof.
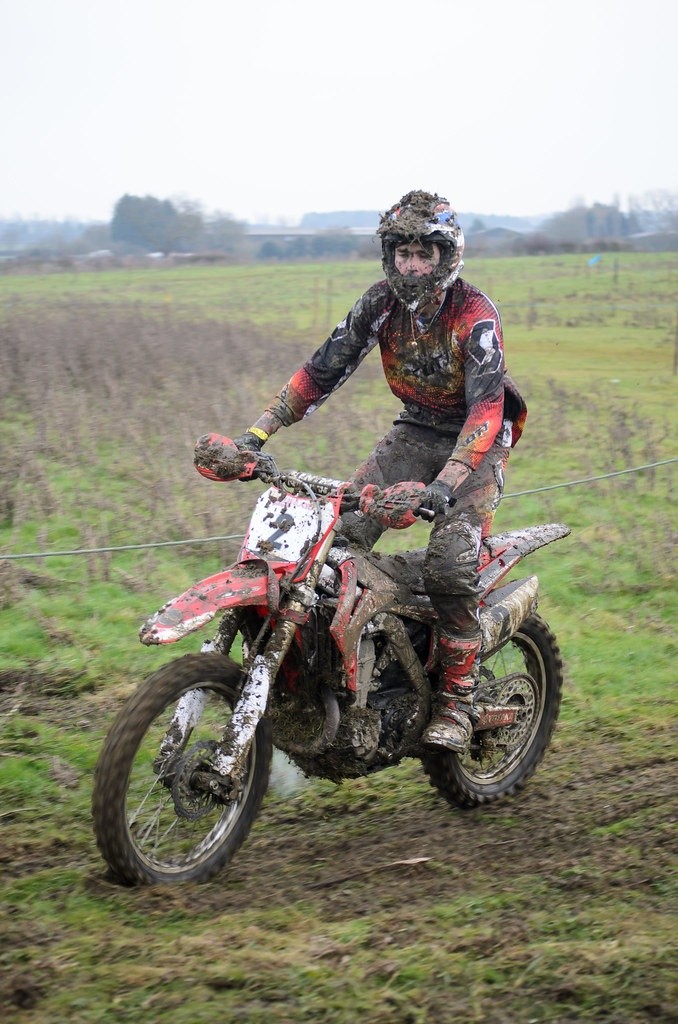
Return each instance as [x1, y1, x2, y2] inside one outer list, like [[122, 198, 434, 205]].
[[423, 630, 482, 755]]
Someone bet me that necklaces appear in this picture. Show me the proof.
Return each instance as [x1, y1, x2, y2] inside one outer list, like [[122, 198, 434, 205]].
[[410, 289, 446, 348]]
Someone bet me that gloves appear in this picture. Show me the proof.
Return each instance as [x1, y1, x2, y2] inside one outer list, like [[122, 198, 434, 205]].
[[232, 431, 266, 481], [419, 479, 455, 523]]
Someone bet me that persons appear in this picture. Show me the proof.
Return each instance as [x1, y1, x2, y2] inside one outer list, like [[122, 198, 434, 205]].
[[232, 189, 526, 754]]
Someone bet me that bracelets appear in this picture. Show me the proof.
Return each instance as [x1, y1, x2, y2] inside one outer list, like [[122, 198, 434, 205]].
[[248, 427, 269, 442]]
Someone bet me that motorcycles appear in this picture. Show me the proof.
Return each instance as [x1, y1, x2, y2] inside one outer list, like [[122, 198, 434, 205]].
[[93, 447, 572, 890]]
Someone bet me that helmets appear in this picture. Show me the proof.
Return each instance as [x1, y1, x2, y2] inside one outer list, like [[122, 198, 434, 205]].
[[378, 196, 465, 312]]
